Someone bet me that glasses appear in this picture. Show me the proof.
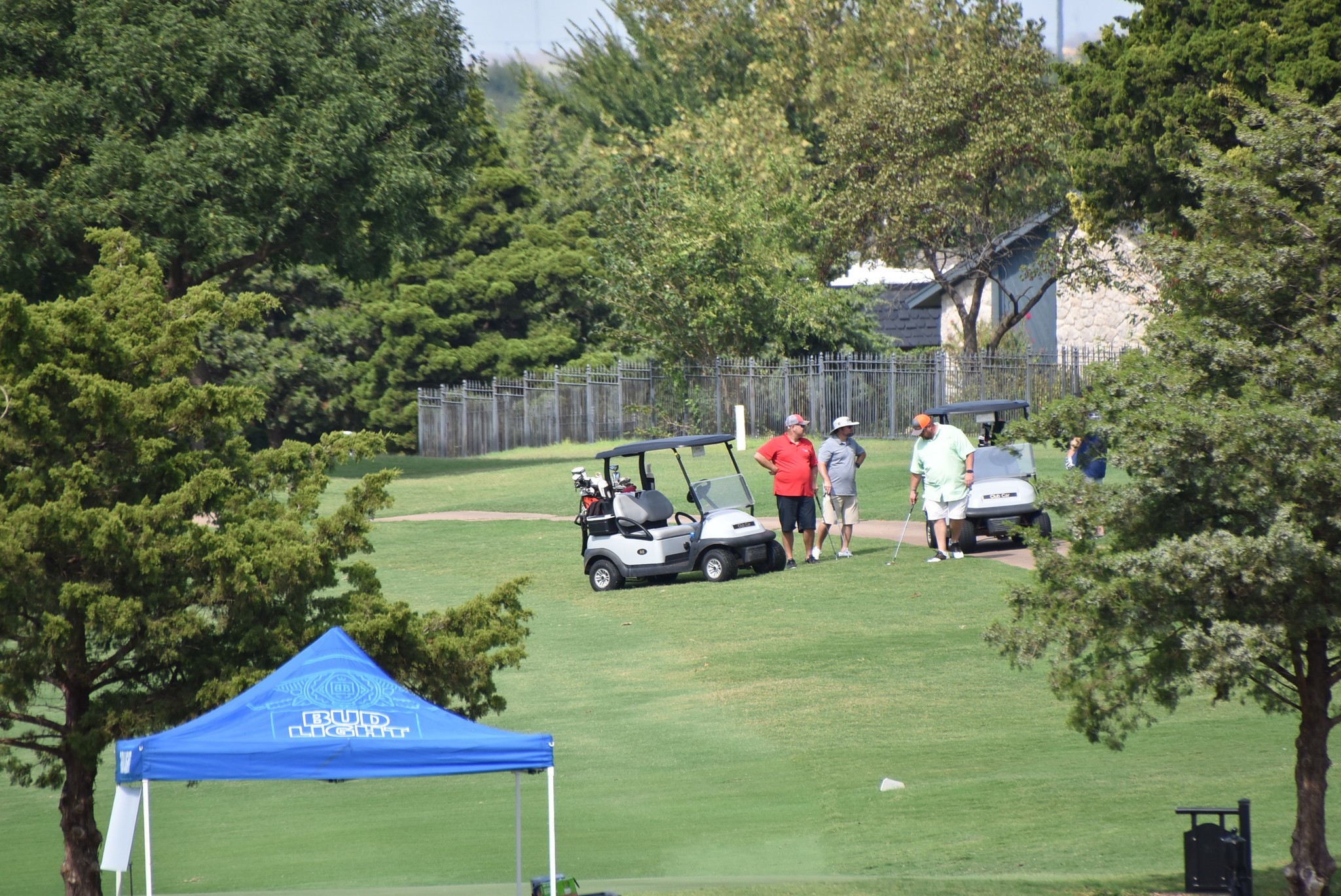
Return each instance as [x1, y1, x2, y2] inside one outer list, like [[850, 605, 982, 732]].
[[847, 426, 853, 429], [922, 426, 928, 433], [794, 424, 805, 428]]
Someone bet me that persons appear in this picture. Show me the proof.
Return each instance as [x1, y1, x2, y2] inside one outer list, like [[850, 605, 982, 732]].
[[753, 414, 819, 570], [1066, 409, 1108, 543], [909, 414, 976, 562], [812, 417, 866, 560]]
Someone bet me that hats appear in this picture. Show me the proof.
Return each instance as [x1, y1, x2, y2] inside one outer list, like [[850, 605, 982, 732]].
[[912, 414, 931, 436], [785, 414, 810, 426], [1089, 413, 1103, 421], [829, 417, 860, 435]]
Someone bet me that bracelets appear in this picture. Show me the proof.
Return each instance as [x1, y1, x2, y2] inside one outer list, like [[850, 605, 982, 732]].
[[855, 463, 860, 468], [911, 489, 917, 492], [965, 470, 974, 474]]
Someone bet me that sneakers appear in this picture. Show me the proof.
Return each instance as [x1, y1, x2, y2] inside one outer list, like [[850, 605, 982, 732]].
[[928, 550, 950, 562], [839, 550, 853, 557], [786, 559, 797, 569], [805, 555, 819, 564], [948, 539, 964, 558], [812, 546, 822, 560]]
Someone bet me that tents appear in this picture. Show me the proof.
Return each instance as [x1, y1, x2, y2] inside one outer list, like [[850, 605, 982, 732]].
[[114, 628, 559, 896]]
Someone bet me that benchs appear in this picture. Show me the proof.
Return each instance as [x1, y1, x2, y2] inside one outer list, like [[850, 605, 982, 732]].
[[613, 490, 701, 541]]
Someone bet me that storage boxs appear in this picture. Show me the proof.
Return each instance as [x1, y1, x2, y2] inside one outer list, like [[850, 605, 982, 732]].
[[587, 514, 619, 536], [530, 875, 581, 896]]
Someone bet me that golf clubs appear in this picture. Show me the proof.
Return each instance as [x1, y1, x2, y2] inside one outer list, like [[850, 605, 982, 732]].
[[609, 464, 637, 491], [829, 492, 850, 558], [886, 491, 918, 565], [814, 492, 838, 560]]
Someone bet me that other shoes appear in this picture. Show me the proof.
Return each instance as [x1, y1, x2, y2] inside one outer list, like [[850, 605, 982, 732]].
[[1075, 534, 1105, 540]]
